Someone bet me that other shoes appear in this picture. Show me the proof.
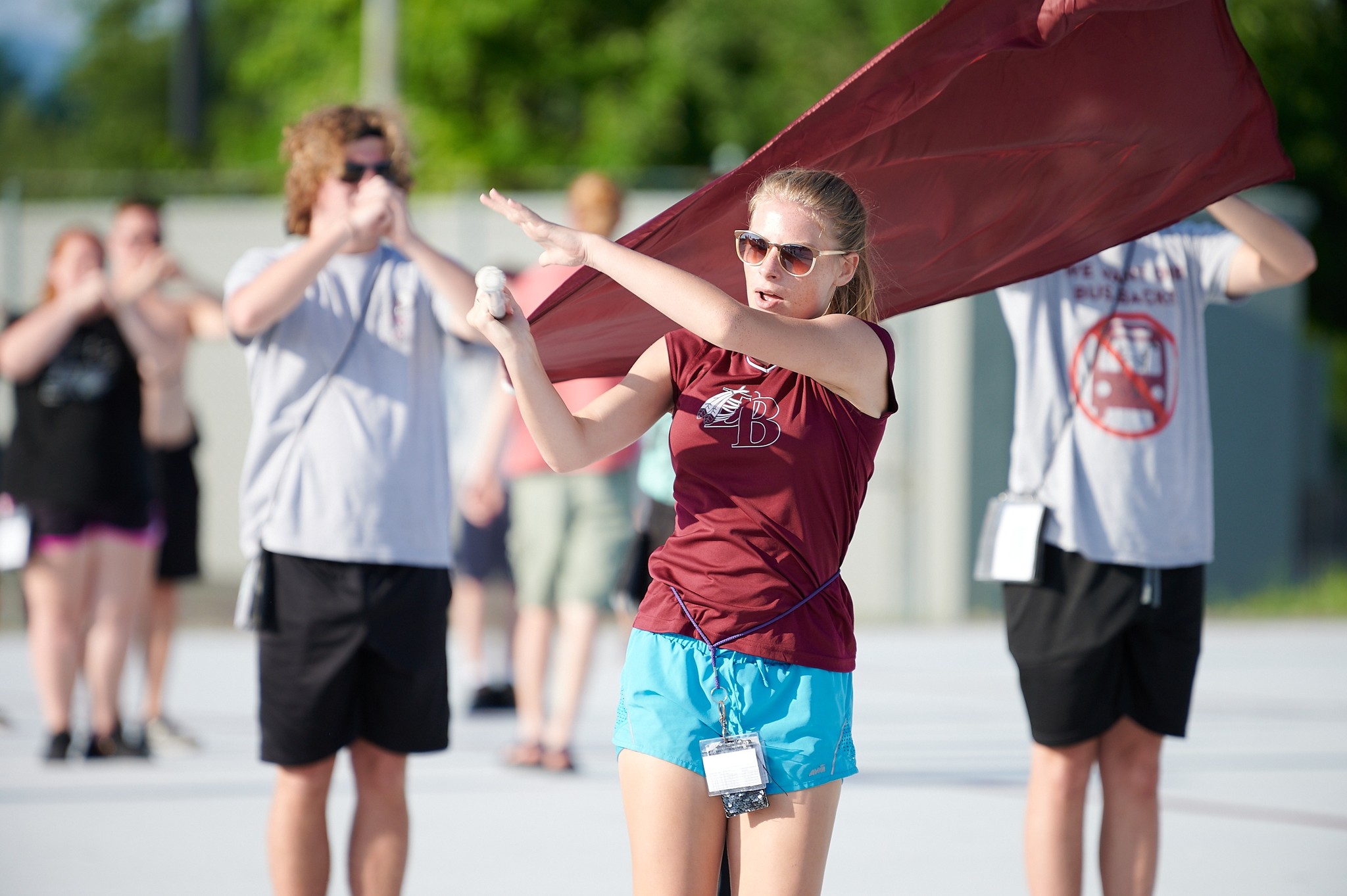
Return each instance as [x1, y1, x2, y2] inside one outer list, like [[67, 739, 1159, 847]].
[[86, 725, 133, 757], [474, 683, 514, 710], [136, 716, 193, 759], [542, 746, 575, 771], [43, 732, 71, 758], [515, 742, 542, 764]]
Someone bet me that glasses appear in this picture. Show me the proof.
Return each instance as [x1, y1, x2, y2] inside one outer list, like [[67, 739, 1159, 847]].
[[339, 161, 391, 185], [734, 229, 852, 277]]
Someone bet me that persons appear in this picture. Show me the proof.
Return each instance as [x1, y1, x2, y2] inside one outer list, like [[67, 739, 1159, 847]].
[[997, 194, 1316, 896], [0, 198, 228, 761], [446, 170, 897, 896], [226, 107, 493, 896]]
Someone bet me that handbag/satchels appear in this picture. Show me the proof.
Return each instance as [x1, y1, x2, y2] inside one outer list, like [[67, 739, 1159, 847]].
[[233, 550, 269, 630], [974, 494, 1051, 586]]
